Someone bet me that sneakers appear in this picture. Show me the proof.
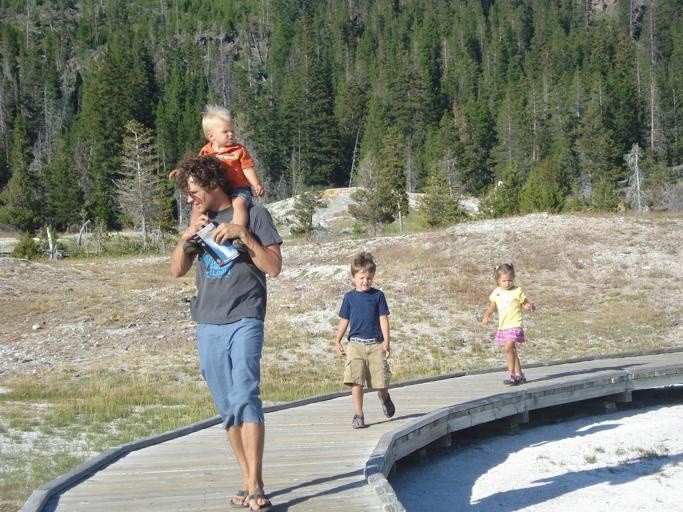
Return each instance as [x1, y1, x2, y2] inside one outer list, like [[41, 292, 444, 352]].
[[180, 241, 204, 256], [351, 414, 364, 428], [378, 390, 395, 418], [502, 372, 527, 385], [231, 239, 247, 254]]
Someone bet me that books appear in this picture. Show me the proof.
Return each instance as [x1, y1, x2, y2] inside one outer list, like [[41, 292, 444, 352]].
[[190, 223, 240, 268]]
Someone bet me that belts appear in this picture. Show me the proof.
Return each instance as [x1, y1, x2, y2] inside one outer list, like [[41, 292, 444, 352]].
[[348, 336, 384, 344]]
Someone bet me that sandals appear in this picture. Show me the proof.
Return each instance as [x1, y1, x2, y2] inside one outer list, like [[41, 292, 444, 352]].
[[228, 489, 250, 508], [245, 493, 272, 512]]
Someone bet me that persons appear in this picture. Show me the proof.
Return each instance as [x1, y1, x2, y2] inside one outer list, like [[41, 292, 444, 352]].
[[168, 101, 264, 253], [480, 262, 535, 386], [334, 252, 395, 430], [168, 148, 283, 511]]
